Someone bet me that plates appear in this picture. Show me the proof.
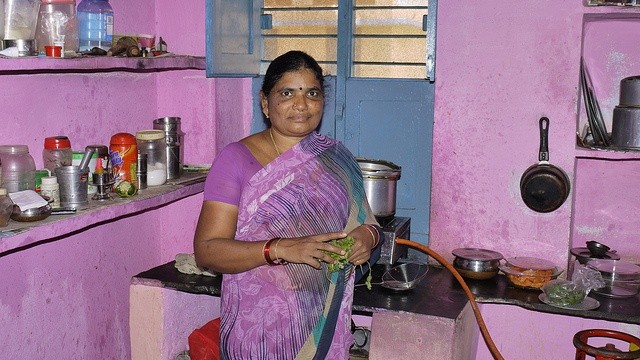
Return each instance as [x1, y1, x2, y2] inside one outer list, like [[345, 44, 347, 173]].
[[569, 247, 621, 263], [539, 292, 601, 311]]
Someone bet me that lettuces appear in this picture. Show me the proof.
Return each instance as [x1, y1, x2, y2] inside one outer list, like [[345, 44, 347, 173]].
[[320, 234, 357, 274]]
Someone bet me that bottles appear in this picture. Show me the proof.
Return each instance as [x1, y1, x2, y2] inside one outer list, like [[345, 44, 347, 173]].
[[0, 144, 37, 194], [85, 144, 113, 193], [1, 188, 13, 228], [69, 151, 100, 195], [42, 135, 72, 175], [135, 129, 167, 186], [108, 132, 140, 191], [40, 175, 62, 209]]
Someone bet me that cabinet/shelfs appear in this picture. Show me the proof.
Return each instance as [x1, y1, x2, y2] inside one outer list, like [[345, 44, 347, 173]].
[[575, 1, 640, 161], [0, 51, 219, 256]]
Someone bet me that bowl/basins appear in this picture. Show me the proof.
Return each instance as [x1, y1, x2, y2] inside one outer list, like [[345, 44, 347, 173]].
[[585, 260, 640, 298], [44, 45, 62, 58], [451, 248, 503, 280], [497, 257, 565, 289]]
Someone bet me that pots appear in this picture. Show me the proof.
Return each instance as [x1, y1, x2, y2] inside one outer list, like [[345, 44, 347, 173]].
[[519, 116, 572, 214], [352, 156, 402, 218]]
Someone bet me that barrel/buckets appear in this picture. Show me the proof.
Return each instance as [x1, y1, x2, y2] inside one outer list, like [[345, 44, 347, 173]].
[[77, 2, 114, 53]]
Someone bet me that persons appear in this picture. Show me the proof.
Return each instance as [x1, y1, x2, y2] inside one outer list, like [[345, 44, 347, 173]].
[[194, 52, 384, 360]]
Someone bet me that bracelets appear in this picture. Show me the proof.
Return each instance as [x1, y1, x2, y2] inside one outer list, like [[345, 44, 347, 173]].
[[264, 238, 282, 266], [263, 240, 271, 266], [359, 226, 375, 247], [364, 224, 380, 249], [275, 238, 289, 265]]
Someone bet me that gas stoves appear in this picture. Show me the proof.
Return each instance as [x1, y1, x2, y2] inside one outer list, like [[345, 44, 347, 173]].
[[376, 214, 411, 264]]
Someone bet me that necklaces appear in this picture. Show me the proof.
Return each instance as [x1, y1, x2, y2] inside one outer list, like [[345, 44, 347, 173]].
[[268, 128, 281, 157]]
